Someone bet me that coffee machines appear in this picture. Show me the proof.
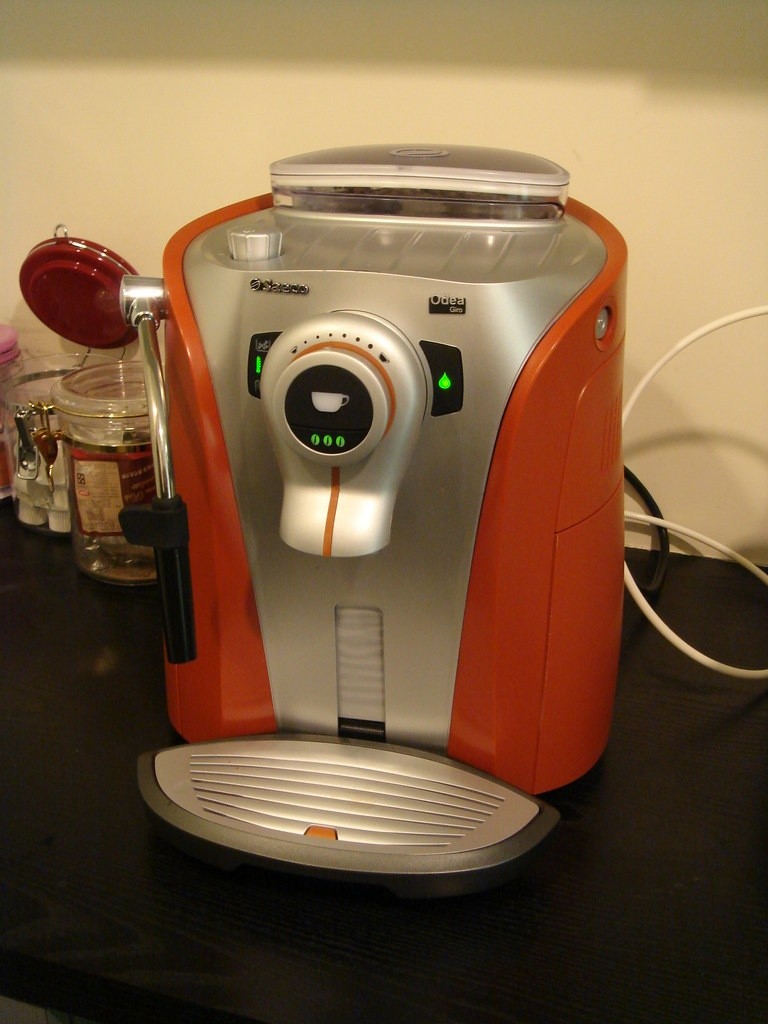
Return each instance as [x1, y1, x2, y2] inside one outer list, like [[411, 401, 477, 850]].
[[131, 142, 624, 877]]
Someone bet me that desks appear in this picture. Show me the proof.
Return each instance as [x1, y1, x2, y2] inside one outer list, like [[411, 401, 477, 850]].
[[0, 494, 768, 1024]]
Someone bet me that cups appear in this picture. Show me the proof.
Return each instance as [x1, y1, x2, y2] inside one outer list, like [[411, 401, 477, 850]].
[[0, 324, 27, 501], [54, 363, 157, 582]]
